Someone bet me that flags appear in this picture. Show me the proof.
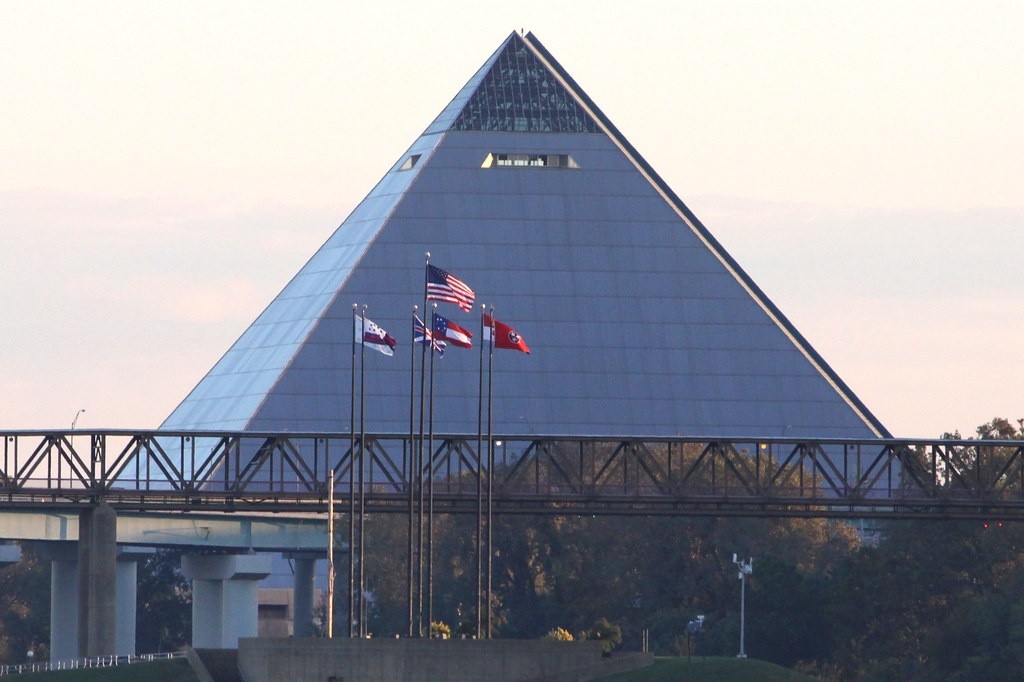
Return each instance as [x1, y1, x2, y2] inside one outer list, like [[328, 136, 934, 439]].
[[435, 312, 472, 349], [414, 316, 447, 359], [364, 318, 396, 356], [484, 313, 498, 342], [494, 320, 530, 354], [427, 263, 475, 314], [355, 315, 362, 343]]
